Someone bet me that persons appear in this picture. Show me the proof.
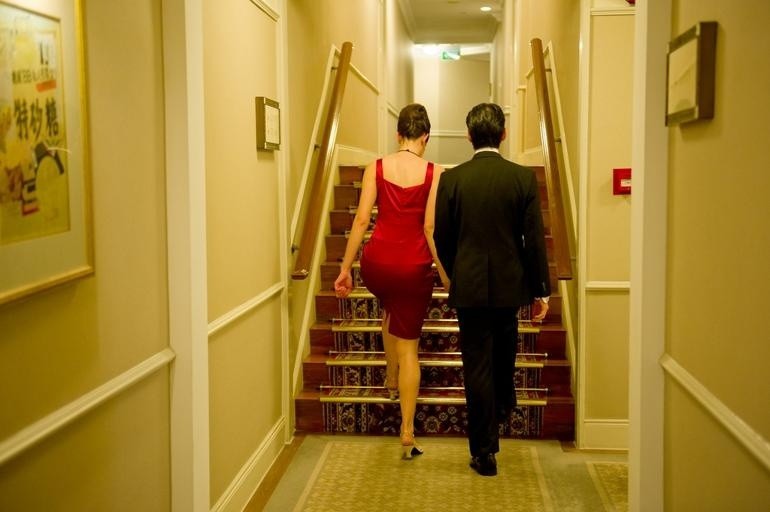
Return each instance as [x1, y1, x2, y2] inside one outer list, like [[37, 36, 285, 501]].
[[334, 104, 451, 460], [433, 102, 552, 477]]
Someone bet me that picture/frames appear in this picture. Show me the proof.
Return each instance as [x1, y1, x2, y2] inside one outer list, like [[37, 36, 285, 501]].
[[0, 0, 96, 309]]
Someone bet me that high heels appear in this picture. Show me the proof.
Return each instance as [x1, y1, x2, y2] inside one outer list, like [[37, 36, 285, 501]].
[[385, 380, 403, 401], [400, 436, 426, 461]]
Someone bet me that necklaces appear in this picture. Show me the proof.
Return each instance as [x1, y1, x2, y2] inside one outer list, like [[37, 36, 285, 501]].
[[398, 149, 420, 157]]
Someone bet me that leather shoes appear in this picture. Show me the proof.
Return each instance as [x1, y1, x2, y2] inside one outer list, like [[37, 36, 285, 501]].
[[498, 389, 518, 424], [467, 451, 500, 477]]
